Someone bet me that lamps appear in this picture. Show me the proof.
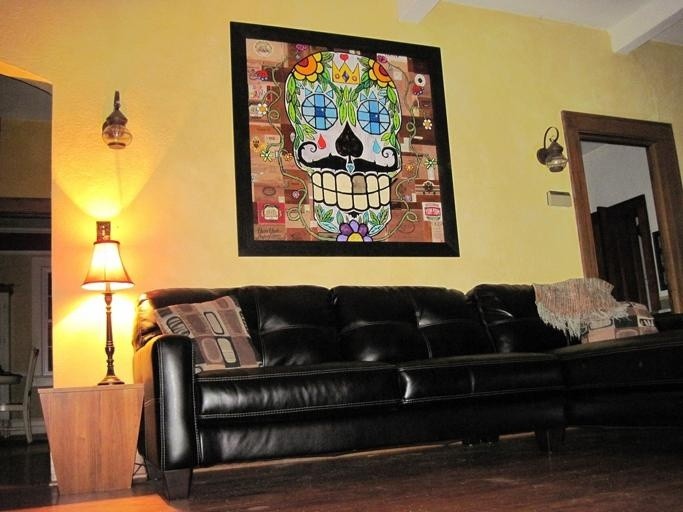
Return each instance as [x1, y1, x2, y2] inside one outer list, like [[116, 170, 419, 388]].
[[537, 126, 568, 171], [100, 90, 133, 150], [81, 220, 134, 385]]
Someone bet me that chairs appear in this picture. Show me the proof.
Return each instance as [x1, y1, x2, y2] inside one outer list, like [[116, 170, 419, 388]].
[[0, 345, 40, 444]]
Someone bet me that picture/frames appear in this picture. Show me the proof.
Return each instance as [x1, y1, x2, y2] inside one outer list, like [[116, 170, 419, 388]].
[[227, 21, 462, 259]]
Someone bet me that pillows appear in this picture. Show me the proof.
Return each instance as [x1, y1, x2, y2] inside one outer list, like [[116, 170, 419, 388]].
[[578, 301, 660, 344], [149, 296, 263, 376]]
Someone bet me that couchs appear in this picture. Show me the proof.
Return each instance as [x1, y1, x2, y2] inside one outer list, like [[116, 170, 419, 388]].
[[131, 277, 675, 500]]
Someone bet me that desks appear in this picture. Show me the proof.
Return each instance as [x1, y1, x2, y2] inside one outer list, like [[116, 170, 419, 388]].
[[36, 383, 144, 494]]
